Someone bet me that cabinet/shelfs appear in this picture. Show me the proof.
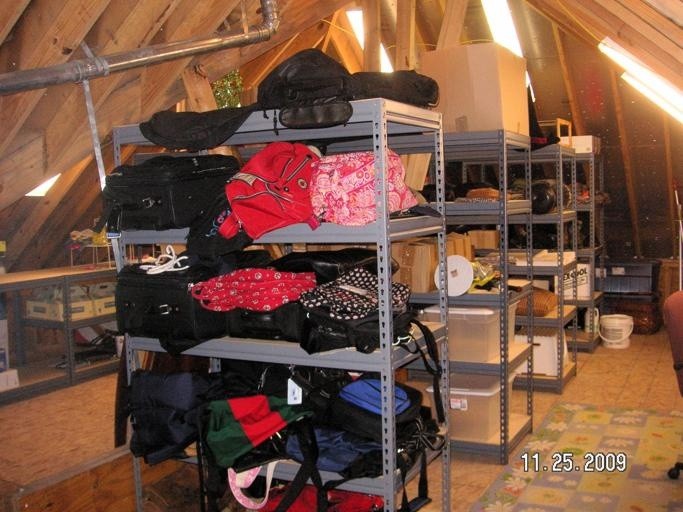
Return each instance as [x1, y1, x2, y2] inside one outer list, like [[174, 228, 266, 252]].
[[461, 145, 577, 396], [406, 128, 533, 466], [459, 153, 597, 354], [111, 97, 450, 511]]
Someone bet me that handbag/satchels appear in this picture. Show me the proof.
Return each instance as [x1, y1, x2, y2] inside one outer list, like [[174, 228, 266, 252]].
[[192, 248, 419, 355], [125, 356, 439, 512], [351, 70, 439, 109], [255, 48, 359, 130], [186, 185, 254, 268], [225, 140, 322, 240], [309, 147, 442, 227]]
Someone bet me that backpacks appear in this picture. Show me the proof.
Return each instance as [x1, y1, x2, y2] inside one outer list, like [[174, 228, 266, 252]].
[[102, 153, 239, 232]]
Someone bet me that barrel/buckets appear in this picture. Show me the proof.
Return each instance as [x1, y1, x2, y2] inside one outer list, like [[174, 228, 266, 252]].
[[598, 312, 634, 350], [583, 308, 600, 334]]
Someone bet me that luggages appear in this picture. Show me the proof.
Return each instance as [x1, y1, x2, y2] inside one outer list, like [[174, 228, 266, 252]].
[[116, 250, 266, 353]]
